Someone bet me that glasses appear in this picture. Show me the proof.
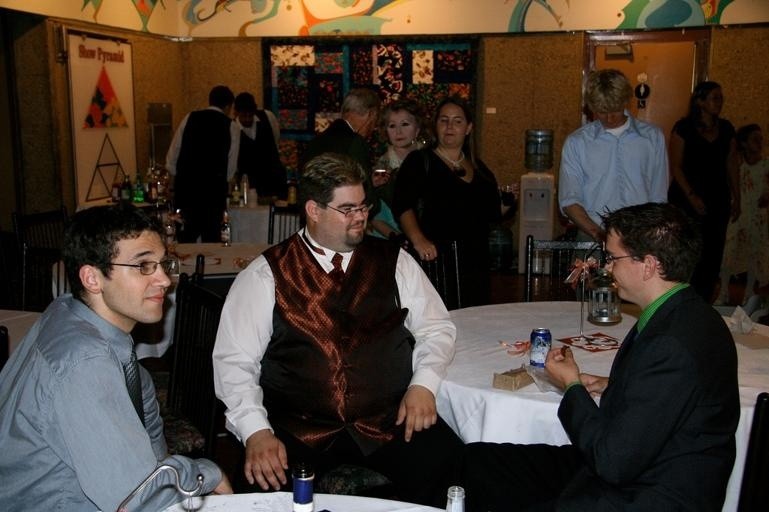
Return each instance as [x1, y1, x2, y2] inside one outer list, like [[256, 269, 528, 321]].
[[312, 194, 375, 219], [603, 247, 647, 265], [89, 255, 179, 281]]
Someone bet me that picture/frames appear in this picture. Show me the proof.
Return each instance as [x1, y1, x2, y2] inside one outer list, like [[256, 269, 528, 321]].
[[260, 33, 481, 181]]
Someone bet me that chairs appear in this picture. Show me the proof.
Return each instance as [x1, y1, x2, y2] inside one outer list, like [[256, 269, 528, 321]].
[[1, 325, 10, 368], [525, 235, 609, 303], [391, 233, 464, 310], [736, 392, 769, 512], [164, 253, 237, 463], [14, 207, 76, 312]]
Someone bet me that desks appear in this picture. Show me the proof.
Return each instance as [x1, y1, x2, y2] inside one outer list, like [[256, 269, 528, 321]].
[[163, 492, 445, 512], [2, 309, 41, 355], [414, 302, 769, 446], [165, 206, 304, 282]]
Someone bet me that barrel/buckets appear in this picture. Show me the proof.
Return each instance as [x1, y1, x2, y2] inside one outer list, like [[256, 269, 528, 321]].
[[524, 127, 553, 173]]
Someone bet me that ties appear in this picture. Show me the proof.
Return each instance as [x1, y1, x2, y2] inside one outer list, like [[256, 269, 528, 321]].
[[120, 335, 152, 432], [327, 254, 348, 291]]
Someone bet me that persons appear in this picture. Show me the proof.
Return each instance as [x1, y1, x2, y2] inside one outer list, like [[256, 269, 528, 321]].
[[0, 204, 233, 512], [557, 69, 670, 304], [212, 151, 457, 509], [476, 202, 741, 512], [712, 124, 769, 306], [668, 81, 741, 303]]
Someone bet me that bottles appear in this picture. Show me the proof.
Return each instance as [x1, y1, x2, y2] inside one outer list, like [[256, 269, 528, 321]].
[[290, 464, 316, 512], [445, 485, 466, 512], [232, 173, 258, 210], [112, 170, 159, 204], [220, 211, 232, 247], [287, 178, 298, 205], [164, 213, 176, 250]]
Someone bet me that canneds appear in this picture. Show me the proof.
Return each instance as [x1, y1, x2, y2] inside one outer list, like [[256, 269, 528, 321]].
[[529, 327, 552, 369]]
